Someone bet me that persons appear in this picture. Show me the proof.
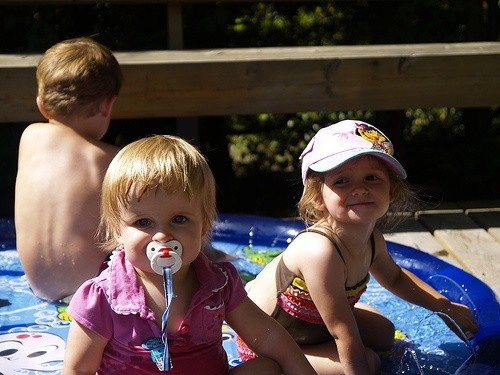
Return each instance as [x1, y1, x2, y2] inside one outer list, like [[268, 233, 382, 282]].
[[15, 37, 124, 302], [64, 137, 316, 375], [237, 119, 481, 375]]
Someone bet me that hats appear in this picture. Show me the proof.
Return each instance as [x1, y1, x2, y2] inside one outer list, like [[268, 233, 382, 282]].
[[298, 118, 407, 186]]
